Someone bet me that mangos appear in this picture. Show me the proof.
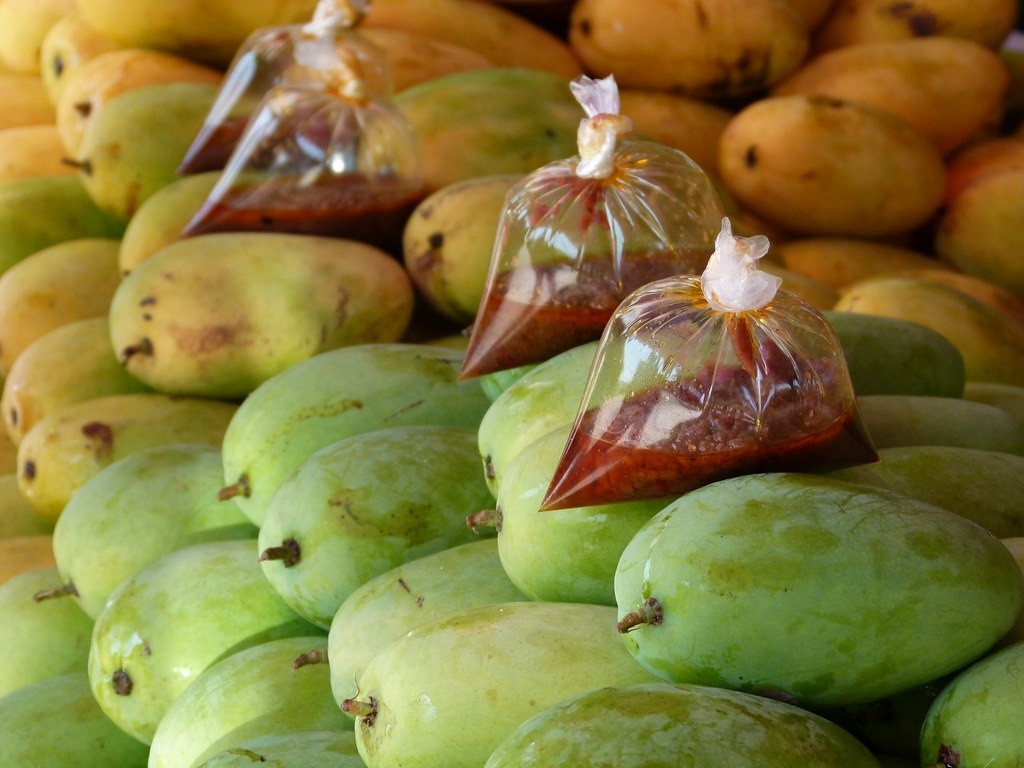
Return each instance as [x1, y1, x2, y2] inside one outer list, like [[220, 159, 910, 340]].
[[0, 0, 1024, 768]]
